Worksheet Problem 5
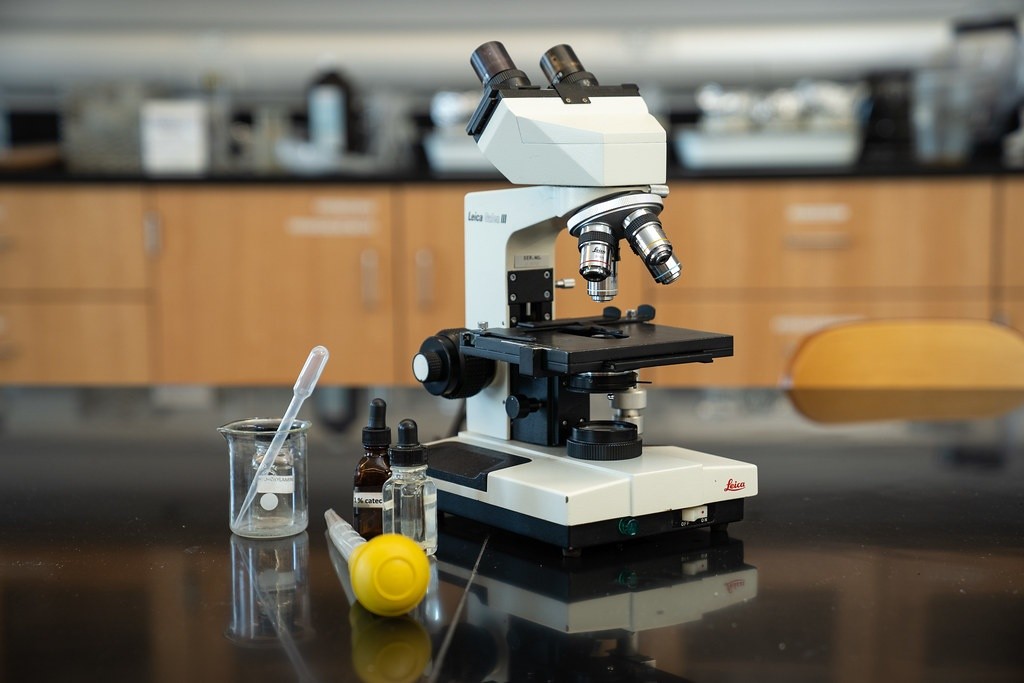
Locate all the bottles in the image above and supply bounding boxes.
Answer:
[353,398,392,539]
[382,419,437,556]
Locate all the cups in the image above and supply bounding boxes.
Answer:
[215,417,312,537]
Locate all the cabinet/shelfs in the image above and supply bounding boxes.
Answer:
[0,175,1024,386]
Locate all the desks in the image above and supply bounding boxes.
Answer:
[0,385,1023,683]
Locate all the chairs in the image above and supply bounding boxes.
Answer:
[786,317,1023,388]
